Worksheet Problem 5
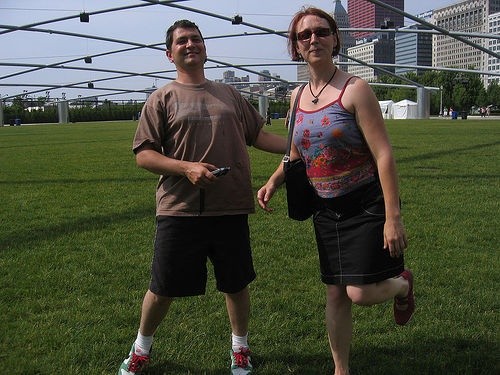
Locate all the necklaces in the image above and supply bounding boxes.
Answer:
[309,66,337,103]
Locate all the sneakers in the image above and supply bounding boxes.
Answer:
[394,271,415,326]
[230,345,253,375]
[118,343,152,375]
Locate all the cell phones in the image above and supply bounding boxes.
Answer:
[211,166,230,176]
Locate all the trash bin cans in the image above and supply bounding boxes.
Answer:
[9,119,14,126]
[72,118,75,123]
[271,113,278,119]
[452,111,457,119]
[461,111,467,119]
[16,119,21,126]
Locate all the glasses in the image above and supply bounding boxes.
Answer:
[297,28,332,40]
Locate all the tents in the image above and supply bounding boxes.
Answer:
[391,99,418,119]
[378,100,393,119]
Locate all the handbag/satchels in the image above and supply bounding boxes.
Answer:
[282,157,317,220]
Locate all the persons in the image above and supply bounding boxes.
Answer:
[449,107,453,117]
[443,106,448,118]
[480,106,490,118]
[116,19,291,375]
[257,7,414,375]
[451,108,457,120]
[137,111,141,120]
[266,108,272,126]
[285,109,290,128]
